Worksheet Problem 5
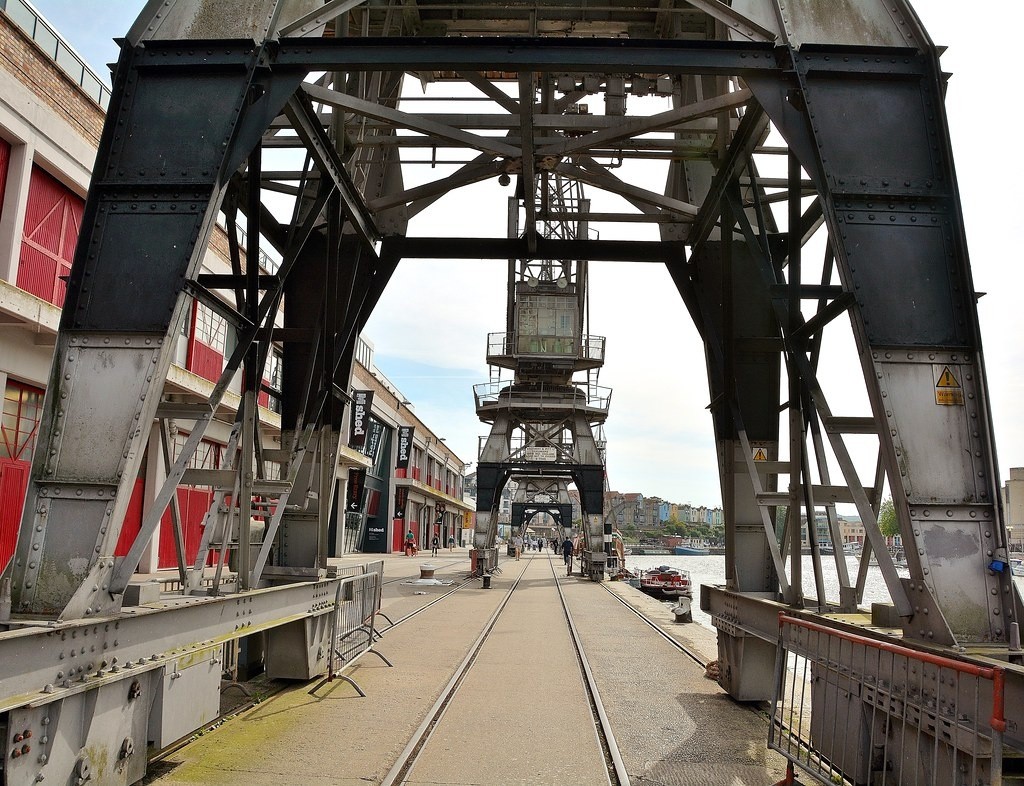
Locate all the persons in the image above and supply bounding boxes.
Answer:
[566,547,573,577]
[524,537,544,552]
[553,537,559,555]
[513,534,523,561]
[497,535,502,550]
[430,532,439,557]
[406,529,414,548]
[562,536,574,566]
[448,535,455,552]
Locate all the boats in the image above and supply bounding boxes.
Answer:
[641,565,692,595]
[673,545,710,556]
